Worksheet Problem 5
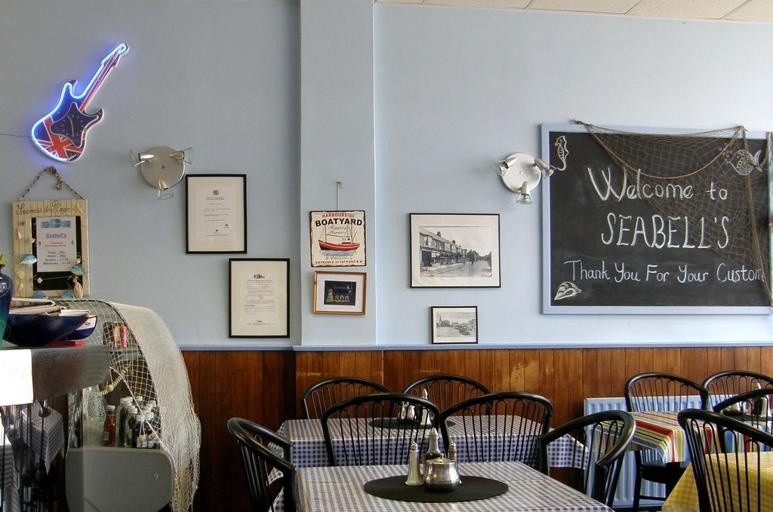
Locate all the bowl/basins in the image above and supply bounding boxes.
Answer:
[2,306,89,349]
[60,315,96,342]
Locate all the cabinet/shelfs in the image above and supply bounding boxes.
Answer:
[53,297,201,510]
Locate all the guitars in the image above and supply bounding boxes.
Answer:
[31,40,128,163]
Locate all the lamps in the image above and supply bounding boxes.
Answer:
[127,145,194,201]
[494,152,554,205]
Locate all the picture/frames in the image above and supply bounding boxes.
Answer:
[428,306,479,344]
[407,210,502,289]
[183,171,248,255]
[227,255,291,338]
[309,208,366,272]
[310,269,366,315]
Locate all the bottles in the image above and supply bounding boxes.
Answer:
[723,382,772,418]
[103,393,162,448]
[0,263,12,350]
[397,387,462,491]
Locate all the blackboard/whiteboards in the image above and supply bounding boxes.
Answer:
[541,122,773,315]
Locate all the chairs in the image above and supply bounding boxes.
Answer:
[401,374,494,415]
[227,417,297,511]
[625,372,709,509]
[426,392,553,469]
[321,392,451,466]
[300,376,402,419]
[0,392,67,512]
[661,370,773,511]
[537,410,636,502]
